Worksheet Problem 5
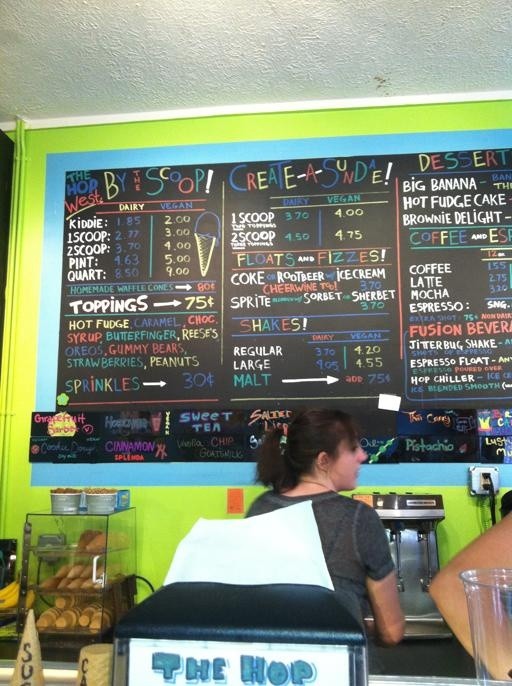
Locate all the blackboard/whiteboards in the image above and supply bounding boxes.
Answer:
[30,149,512,465]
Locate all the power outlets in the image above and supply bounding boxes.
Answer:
[468,465,501,498]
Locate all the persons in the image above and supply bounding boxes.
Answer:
[427,490,512,681]
[245,406,406,647]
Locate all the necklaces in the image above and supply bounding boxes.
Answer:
[300,478,337,492]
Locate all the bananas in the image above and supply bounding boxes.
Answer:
[0,576,36,612]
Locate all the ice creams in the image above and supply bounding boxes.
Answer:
[194,211,221,278]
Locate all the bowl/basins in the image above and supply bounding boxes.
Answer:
[50,491,81,515]
[85,492,118,514]
